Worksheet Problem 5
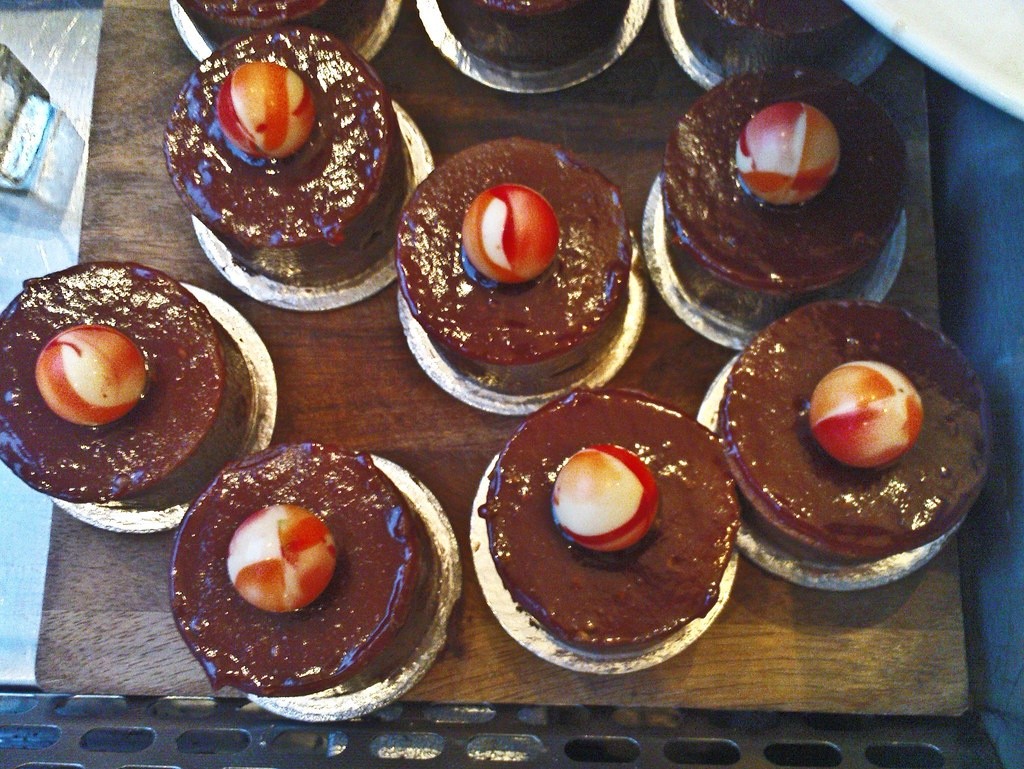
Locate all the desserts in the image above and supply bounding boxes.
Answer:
[0,0,995,723]
